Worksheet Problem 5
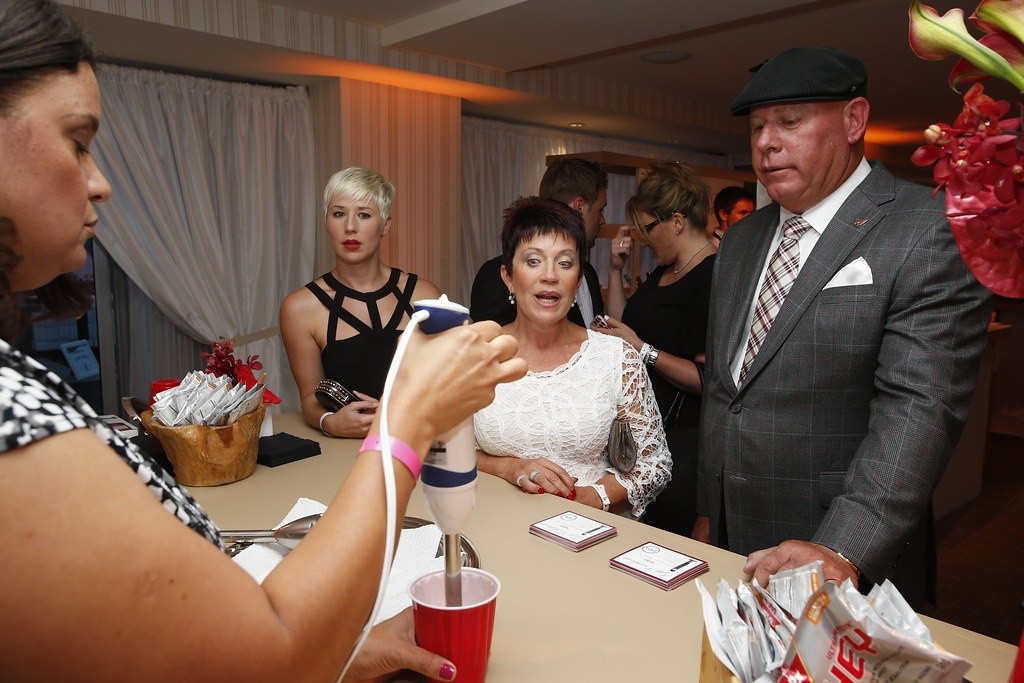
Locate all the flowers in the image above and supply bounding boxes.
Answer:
[908,0,1024,298]
[201,335,262,379]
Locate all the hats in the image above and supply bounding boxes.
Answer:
[730,44,868,117]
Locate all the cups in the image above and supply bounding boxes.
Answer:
[409,566,500,683]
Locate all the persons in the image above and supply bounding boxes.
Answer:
[472,197,673,522]
[691,46,994,613]
[0,0,528,683]
[471,153,609,333]
[609,160,717,524]
[280,166,443,441]
[711,185,759,242]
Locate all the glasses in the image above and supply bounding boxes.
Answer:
[637,210,688,237]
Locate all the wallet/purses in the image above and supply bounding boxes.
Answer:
[315,378,367,415]
[608,419,638,475]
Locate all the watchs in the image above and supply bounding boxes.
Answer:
[646,345,660,368]
[834,549,863,579]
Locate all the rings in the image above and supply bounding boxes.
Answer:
[619,241,623,248]
[516,474,528,486]
[530,470,540,480]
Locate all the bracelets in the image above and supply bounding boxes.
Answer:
[590,482,610,513]
[640,342,651,361]
[358,433,423,488]
[318,412,332,438]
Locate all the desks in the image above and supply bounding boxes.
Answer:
[168,414,1019,683]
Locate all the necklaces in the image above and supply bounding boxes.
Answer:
[673,240,712,274]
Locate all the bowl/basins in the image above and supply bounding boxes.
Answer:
[140,403,266,485]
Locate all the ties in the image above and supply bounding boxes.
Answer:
[736,216,813,394]
